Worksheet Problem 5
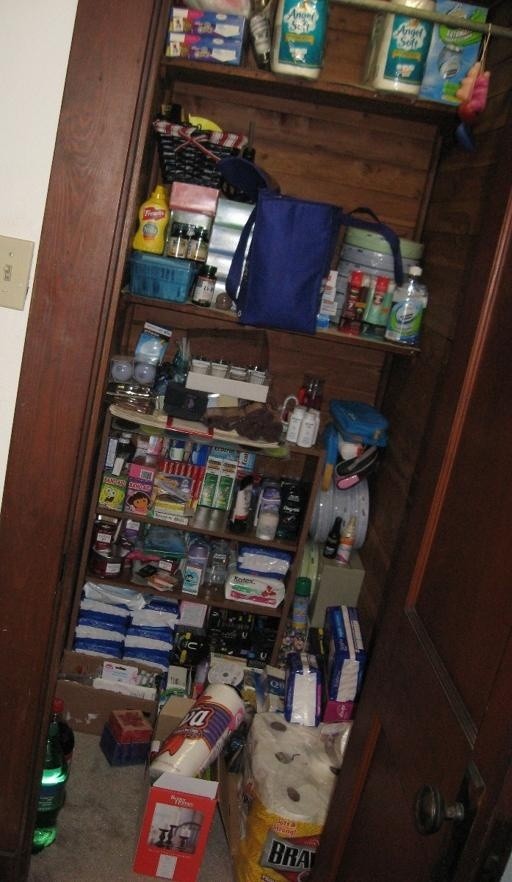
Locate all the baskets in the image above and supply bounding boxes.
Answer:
[153,117,251,191]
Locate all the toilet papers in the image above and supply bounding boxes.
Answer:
[220,713,353,882]
[150,684,246,783]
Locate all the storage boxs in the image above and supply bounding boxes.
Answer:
[133,694,221,882]
[308,550,367,628]
[419,1,489,107]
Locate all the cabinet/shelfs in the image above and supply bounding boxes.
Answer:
[59,405,328,703]
[120,55,476,358]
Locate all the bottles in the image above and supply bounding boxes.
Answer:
[184,225,210,264]
[121,432,135,464]
[285,404,321,449]
[165,221,190,259]
[323,513,358,566]
[255,487,282,541]
[45,697,76,812]
[191,263,217,308]
[185,544,209,585]
[247,1,272,70]
[30,723,69,857]
[115,437,131,464]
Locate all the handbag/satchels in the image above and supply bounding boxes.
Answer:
[225,187,347,336]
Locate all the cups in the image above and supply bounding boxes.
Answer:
[380,263,429,347]
